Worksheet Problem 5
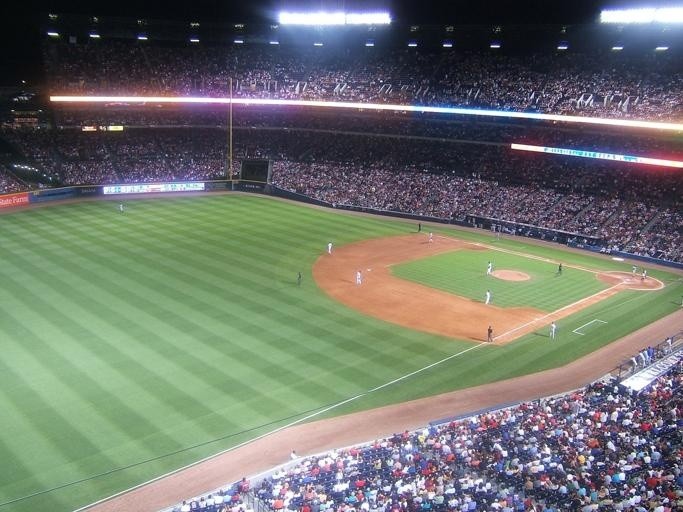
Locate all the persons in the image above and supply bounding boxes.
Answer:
[549,322,556,339]
[487,326,493,342]
[483,290,491,304]
